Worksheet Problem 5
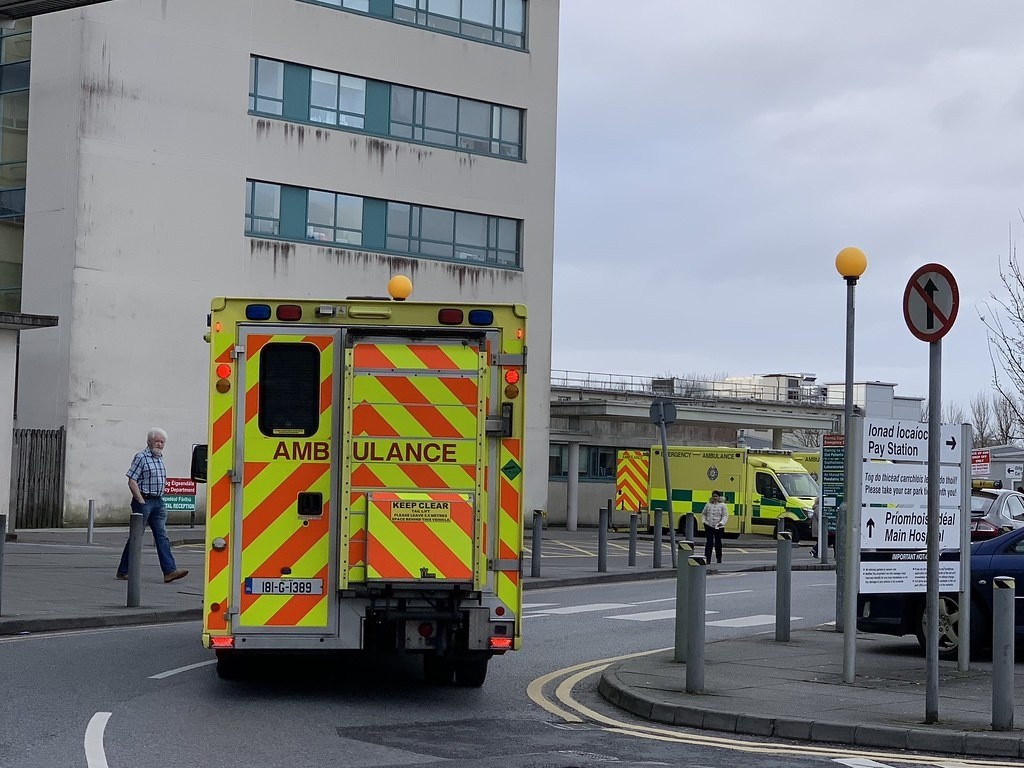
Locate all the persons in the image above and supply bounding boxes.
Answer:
[114,427,189,584]
[701,490,728,565]
[808,496,819,558]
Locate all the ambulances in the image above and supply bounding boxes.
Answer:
[616,446,819,543]
[202,275,530,691]
[791,453,820,487]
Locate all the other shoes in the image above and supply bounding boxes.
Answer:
[707,562,710,564]
[116,573,128,580]
[809,550,818,558]
[164,570,189,583]
[717,559,721,563]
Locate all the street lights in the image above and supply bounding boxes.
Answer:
[835,245,868,683]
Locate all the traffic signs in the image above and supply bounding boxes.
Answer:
[860,507,960,549]
[1006,464,1023,478]
[863,417,962,463]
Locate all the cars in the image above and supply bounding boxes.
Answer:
[857,526,1024,661]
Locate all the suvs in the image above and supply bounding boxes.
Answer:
[971,479,1024,542]
